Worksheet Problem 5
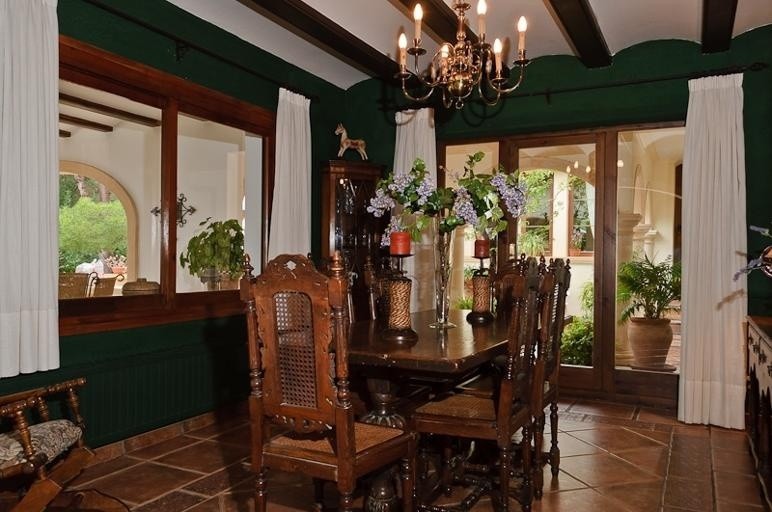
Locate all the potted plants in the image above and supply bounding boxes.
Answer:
[616,255,682,370]
[180,215,243,291]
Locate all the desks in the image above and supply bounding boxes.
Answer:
[320,308,571,509]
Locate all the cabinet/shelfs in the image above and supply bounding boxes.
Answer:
[322,160,388,269]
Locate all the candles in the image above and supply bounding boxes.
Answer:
[389,232,411,255]
[475,239,490,256]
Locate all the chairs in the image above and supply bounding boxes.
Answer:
[59,269,125,299]
[408,253,555,510]
[1,378,97,511]
[242,252,417,510]
[454,257,571,500]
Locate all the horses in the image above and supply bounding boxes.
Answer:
[334,122,368,160]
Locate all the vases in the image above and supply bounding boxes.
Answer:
[427,231,460,328]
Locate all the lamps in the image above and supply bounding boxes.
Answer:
[396,0,531,111]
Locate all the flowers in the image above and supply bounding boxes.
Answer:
[364,150,531,315]
